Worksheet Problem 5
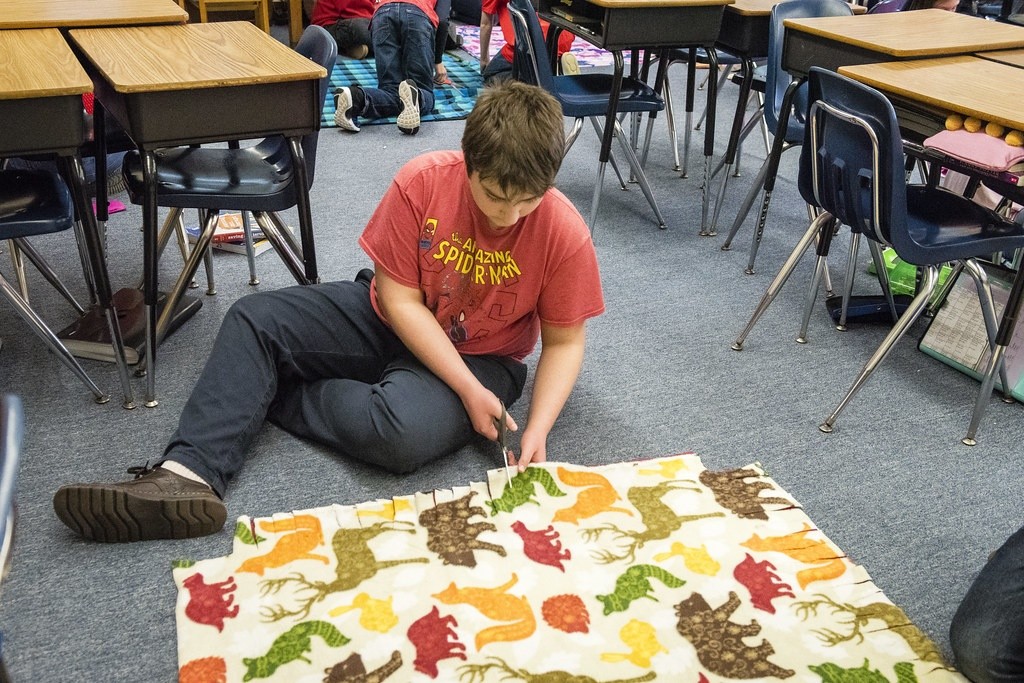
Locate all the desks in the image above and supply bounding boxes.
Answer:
[534,2,736,236]
[746,7,1024,273]
[0,29,133,410]
[191,0,271,34]
[710,0,867,234]
[838,54,1024,329]
[976,48,1024,69]
[69,21,327,408]
[0,2,189,27]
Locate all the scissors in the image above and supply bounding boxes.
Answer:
[433,75,466,96]
[491,401,514,489]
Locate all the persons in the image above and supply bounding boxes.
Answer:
[311,1,377,59]
[331,0,451,137]
[901,0,963,13]
[480,0,583,84]
[5,146,135,199]
[53,76,605,542]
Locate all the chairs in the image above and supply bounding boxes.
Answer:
[123,26,337,297]
[867,0,906,13]
[508,0,668,239]
[635,42,771,178]
[0,166,108,404]
[734,68,1024,433]
[723,1,854,253]
[23,102,133,156]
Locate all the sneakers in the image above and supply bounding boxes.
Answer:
[347,44,369,59]
[397,79,420,136]
[334,87,360,131]
[558,52,579,75]
[53,467,226,543]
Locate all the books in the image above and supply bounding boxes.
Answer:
[185,211,294,258]
[53,287,204,364]
